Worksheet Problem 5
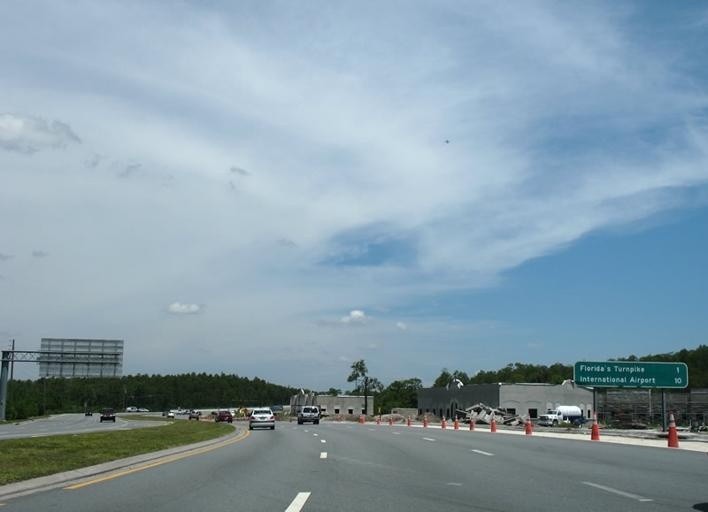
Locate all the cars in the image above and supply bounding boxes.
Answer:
[248,408,275,430]
[100,407,115,423]
[168,411,174,418]
[215,411,233,423]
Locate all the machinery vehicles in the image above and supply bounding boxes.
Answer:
[537,405,583,428]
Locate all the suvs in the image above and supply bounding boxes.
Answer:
[297,405,320,424]
[189,410,199,421]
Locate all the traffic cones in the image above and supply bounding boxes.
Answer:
[667,411,681,448]
[470,416,475,430]
[525,414,534,435]
[454,414,459,431]
[590,411,600,441]
[441,414,447,430]
[423,414,428,428]
[490,413,497,432]
[358,412,412,427]
